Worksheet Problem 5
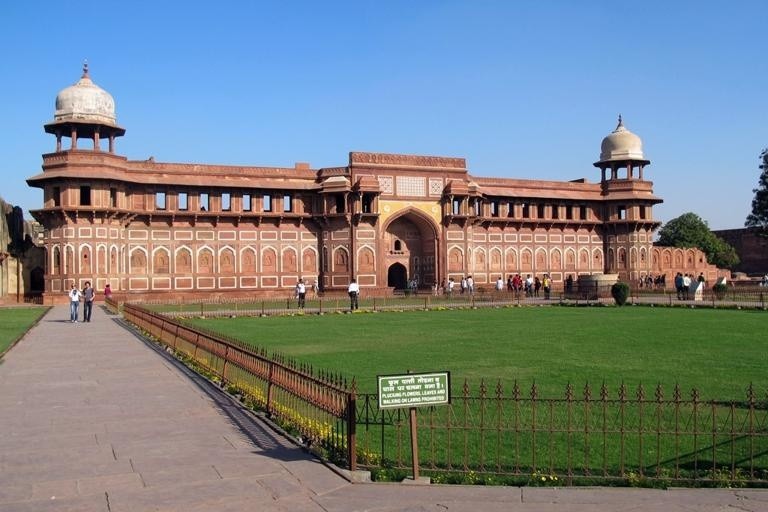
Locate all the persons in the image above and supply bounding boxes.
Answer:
[296,279,306,309]
[103,283,111,307]
[759,274,768,288]
[82,282,96,323]
[68,283,83,323]
[431,275,473,297]
[638,271,707,300]
[494,273,552,300]
[312,280,319,299]
[566,275,574,300]
[348,278,360,310]
[403,278,419,297]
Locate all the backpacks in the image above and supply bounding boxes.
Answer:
[537,282,541,288]
[350,291,356,296]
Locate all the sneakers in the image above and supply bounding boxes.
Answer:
[84,319,91,322]
[71,320,78,323]
